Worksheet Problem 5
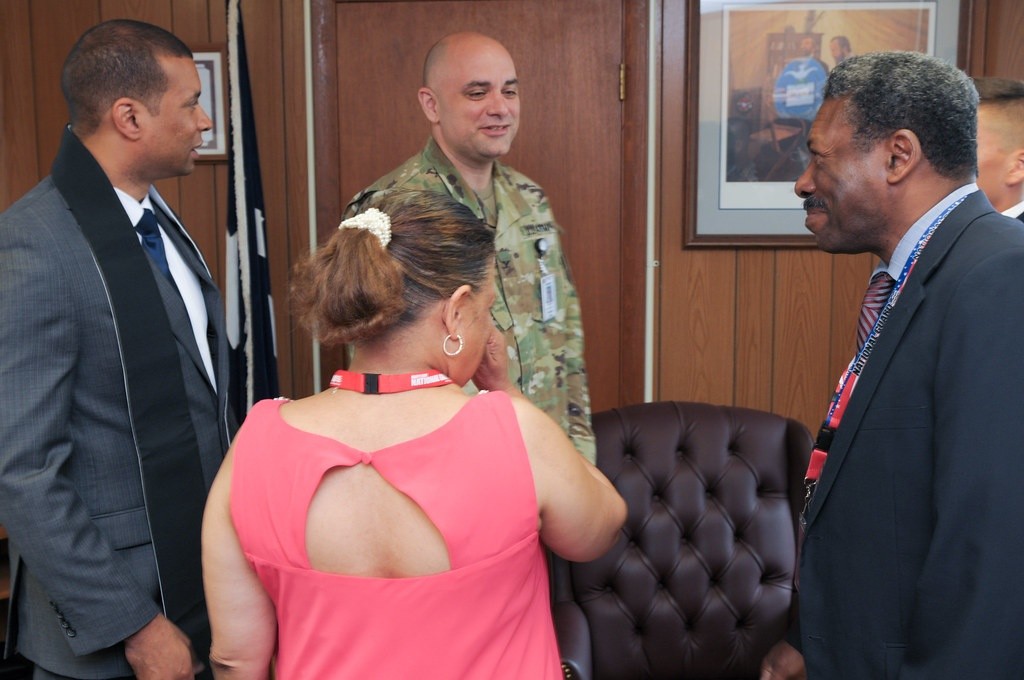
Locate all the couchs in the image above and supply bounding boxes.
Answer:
[543,400,815,680]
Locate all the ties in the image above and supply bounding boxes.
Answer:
[136,207,180,295]
[856,271,897,358]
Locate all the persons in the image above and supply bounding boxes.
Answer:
[0,17,240,680]
[965,76,1024,224]
[758,50,1024,680]
[200,186,628,680]
[341,29,597,474]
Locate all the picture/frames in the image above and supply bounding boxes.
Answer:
[190,42,229,164]
[684,0,975,251]
